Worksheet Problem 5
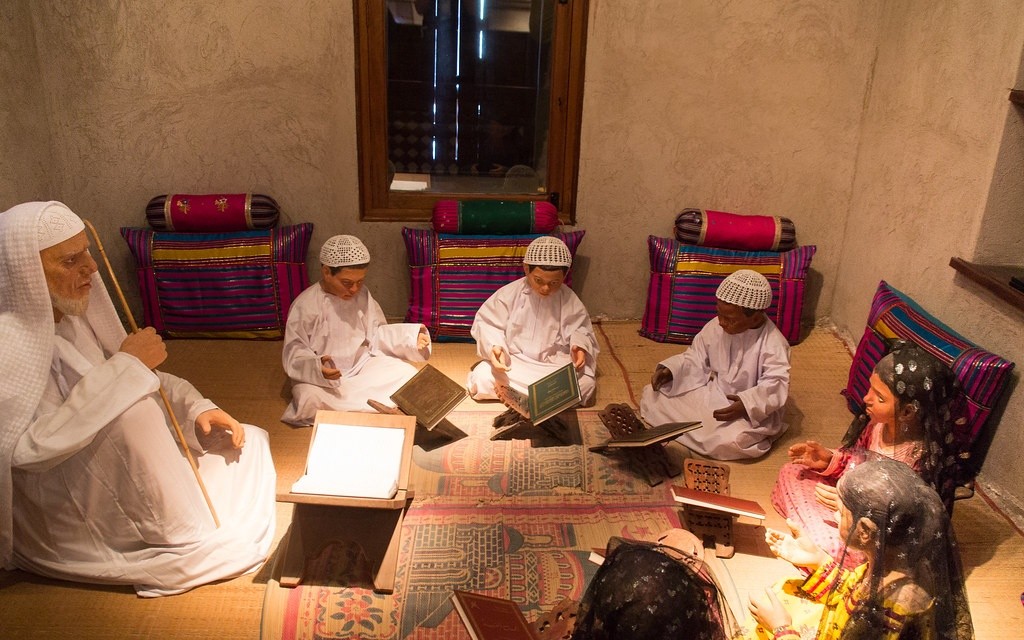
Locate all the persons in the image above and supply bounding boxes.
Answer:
[0,200,277,599]
[639,268,792,461]
[466,235,601,409]
[280,233,432,428]
[571,535,743,640]
[746,338,977,640]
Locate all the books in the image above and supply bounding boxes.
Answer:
[289,423,406,500]
[528,363,583,427]
[389,363,469,432]
[607,421,703,447]
[670,484,767,520]
